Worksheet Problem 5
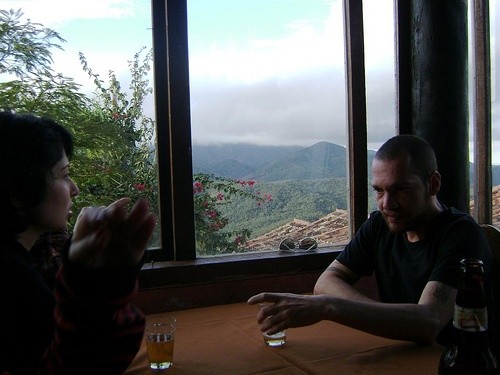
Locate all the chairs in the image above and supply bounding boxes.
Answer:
[480,224,500,371]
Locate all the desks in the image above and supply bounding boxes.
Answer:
[124,292,447,375]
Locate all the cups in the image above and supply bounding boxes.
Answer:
[145,314,177,369]
[258,302,286,346]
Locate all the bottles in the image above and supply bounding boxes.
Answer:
[438,258,497,375]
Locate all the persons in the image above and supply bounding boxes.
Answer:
[247,134,500,375]
[0,110,158,375]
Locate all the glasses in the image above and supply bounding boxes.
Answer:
[279,237,318,253]
